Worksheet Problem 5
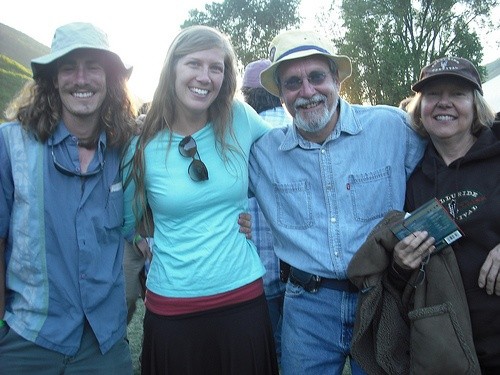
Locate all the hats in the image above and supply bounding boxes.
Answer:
[242,59,272,87]
[412,58,483,97]
[31,22,133,81]
[260,31,353,98]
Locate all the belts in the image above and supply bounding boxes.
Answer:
[288,266,359,294]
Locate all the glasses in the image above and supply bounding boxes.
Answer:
[393,221,431,291]
[178,135,210,183]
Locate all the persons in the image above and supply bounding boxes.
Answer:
[118,114,167,327]
[115,26,280,375]
[246,28,432,375]
[240,58,294,373]
[385,56,500,375]
[1,21,142,375]
